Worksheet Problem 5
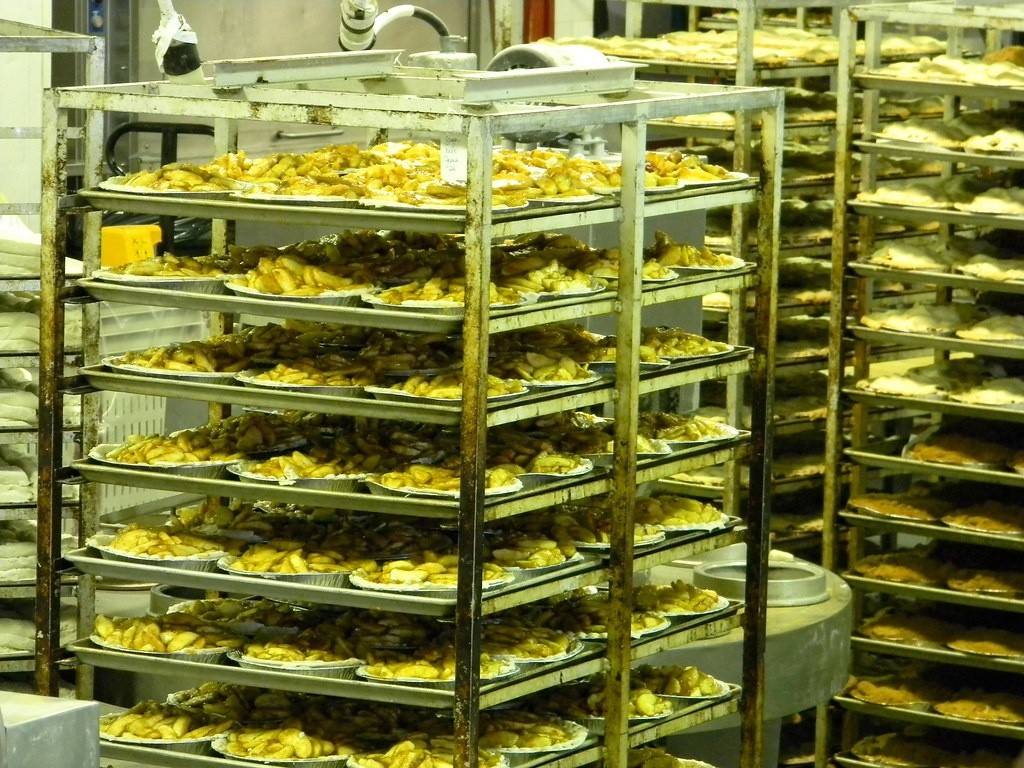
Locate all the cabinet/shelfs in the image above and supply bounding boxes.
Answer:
[492,0,1024,767]
[0,16,108,700]
[31,46,788,768]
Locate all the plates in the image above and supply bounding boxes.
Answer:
[87,0,1024,768]
